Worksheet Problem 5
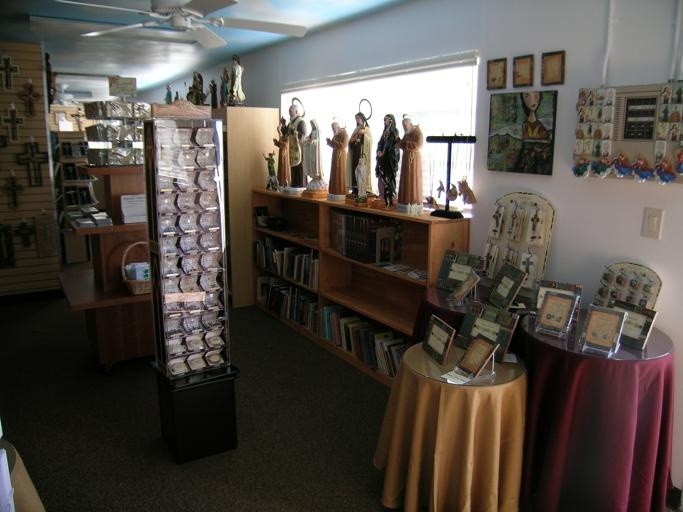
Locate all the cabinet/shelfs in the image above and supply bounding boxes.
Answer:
[250,187,470,389]
[57,107,279,367]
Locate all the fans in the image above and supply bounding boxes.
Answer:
[52,0,307,49]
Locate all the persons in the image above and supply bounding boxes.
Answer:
[519,92,551,140]
[163,55,245,107]
[263,105,423,214]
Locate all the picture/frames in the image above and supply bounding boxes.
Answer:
[513,54,533,87]
[487,57,506,89]
[425,250,657,378]
[542,50,565,84]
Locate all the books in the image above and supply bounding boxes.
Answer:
[255,210,408,379]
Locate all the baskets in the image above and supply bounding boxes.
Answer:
[121,240,153,296]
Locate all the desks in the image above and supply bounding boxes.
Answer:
[372,341,528,512]
[520,302,675,512]
